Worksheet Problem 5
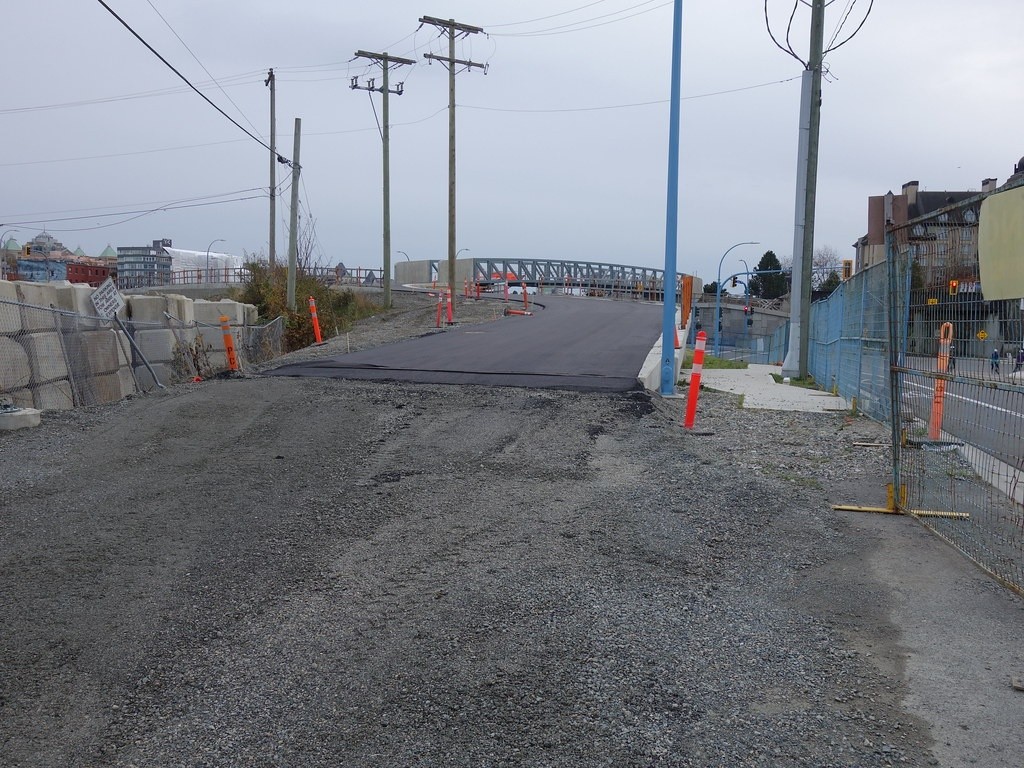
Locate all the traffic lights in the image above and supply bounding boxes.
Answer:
[744,306,747,313]
[732,276,737,287]
[750,307,754,315]
[950,280,959,295]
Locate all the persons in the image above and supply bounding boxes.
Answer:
[1013,350,1024,373]
[989,348,1000,375]
[947,346,956,372]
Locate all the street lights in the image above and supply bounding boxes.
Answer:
[207,238,226,283]
[0,229,23,257]
[4,239,18,280]
[714,241,761,358]
